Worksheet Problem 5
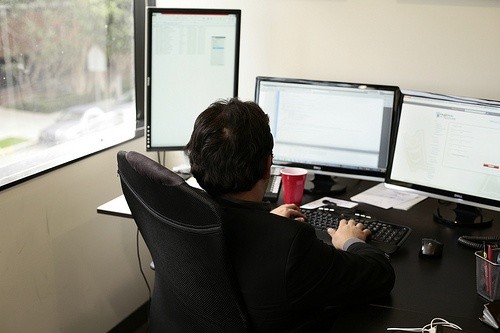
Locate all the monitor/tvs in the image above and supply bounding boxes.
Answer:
[144,8,241,152]
[384,90,500,229]
[254,77,400,195]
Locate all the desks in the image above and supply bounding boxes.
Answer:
[97,176,500,333]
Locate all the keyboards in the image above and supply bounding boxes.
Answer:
[291,208,411,255]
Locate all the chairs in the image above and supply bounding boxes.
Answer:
[117,149,253,333]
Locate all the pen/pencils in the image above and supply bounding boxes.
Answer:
[487,244,493,299]
[482,240,489,295]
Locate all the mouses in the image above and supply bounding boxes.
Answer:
[420,239,444,260]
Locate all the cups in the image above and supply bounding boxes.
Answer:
[280,167,308,206]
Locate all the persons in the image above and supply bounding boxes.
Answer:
[182,97,395,333]
